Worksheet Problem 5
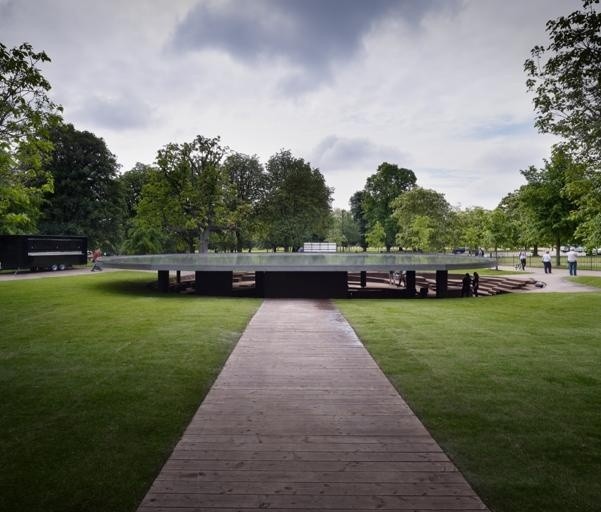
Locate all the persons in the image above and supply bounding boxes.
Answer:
[519,248,527,271]
[461,273,472,297]
[472,272,480,297]
[389,270,407,289]
[90,246,103,272]
[475,247,484,256]
[566,246,580,277]
[542,250,552,274]
